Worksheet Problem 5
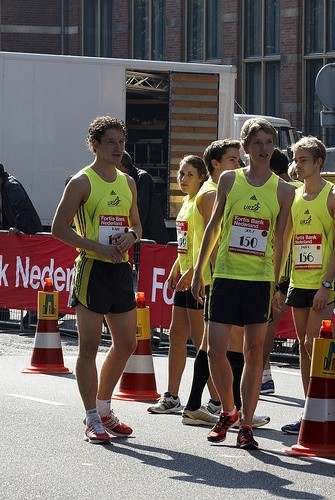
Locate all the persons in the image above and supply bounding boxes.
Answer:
[0,163,42,236]
[259,150,304,393]
[147,156,221,414]
[117,150,170,244]
[190,117,295,447]
[181,138,270,428]
[51,117,142,443]
[279,136,335,434]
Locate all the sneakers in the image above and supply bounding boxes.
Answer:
[237,427,259,449]
[281,418,301,434]
[202,403,223,417]
[182,407,219,425]
[101,413,132,437]
[260,380,275,393]
[207,407,239,442]
[232,411,269,427]
[84,419,110,443]
[148,397,182,412]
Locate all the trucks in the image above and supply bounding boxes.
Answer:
[0,51,301,247]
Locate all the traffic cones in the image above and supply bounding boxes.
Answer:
[19,278,74,375]
[283,320,335,460]
[112,293,162,402]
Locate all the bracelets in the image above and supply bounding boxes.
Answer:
[129,231,138,243]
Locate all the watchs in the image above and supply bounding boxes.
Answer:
[322,281,332,288]
